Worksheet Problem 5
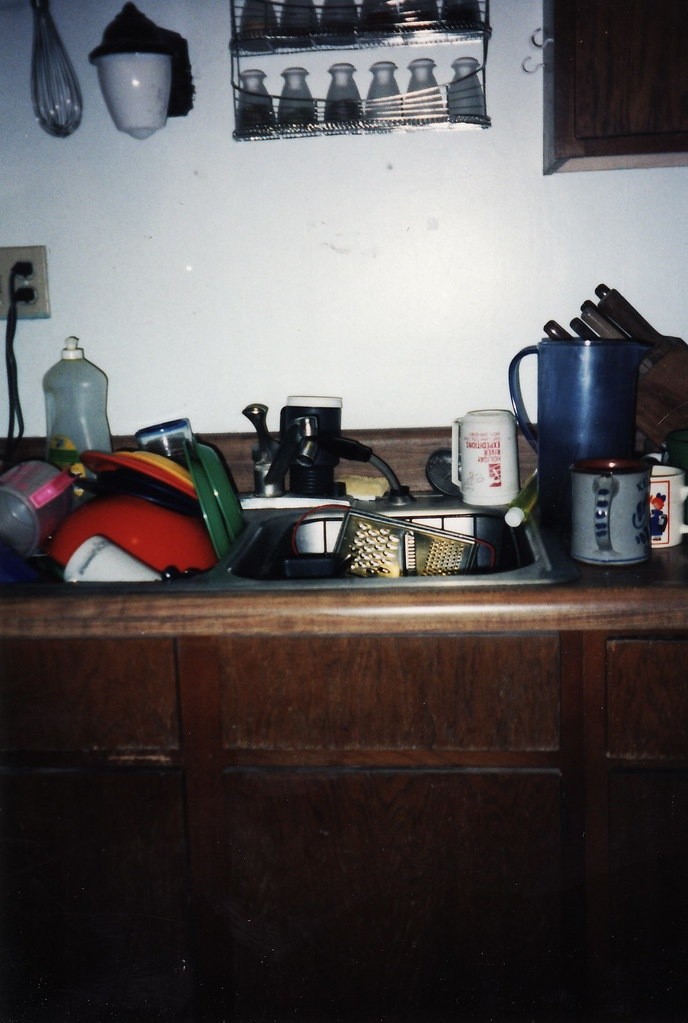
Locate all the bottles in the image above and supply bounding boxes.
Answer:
[43,337,112,471]
[237,2,487,129]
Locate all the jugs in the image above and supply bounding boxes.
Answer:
[508,339,655,568]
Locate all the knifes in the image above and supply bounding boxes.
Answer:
[543,283,671,375]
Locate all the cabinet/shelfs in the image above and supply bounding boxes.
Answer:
[542,0,688,175]
[228,0,492,140]
[0,630,688,1023]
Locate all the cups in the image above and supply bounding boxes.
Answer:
[62,535,162,581]
[0,461,75,559]
[567,456,654,566]
[136,417,196,472]
[279,396,343,500]
[92,51,172,140]
[451,408,519,506]
[647,464,688,548]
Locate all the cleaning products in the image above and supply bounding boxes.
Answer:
[41,335,114,472]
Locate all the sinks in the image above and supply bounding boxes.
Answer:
[0,520,248,582]
[227,510,534,579]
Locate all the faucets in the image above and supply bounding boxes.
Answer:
[242,403,321,498]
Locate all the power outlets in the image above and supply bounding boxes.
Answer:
[0,244,54,320]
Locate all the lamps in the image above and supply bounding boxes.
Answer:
[88,2,196,140]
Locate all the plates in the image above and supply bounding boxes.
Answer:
[79,448,198,502]
[182,438,244,560]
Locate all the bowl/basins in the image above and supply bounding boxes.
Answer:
[46,492,217,574]
